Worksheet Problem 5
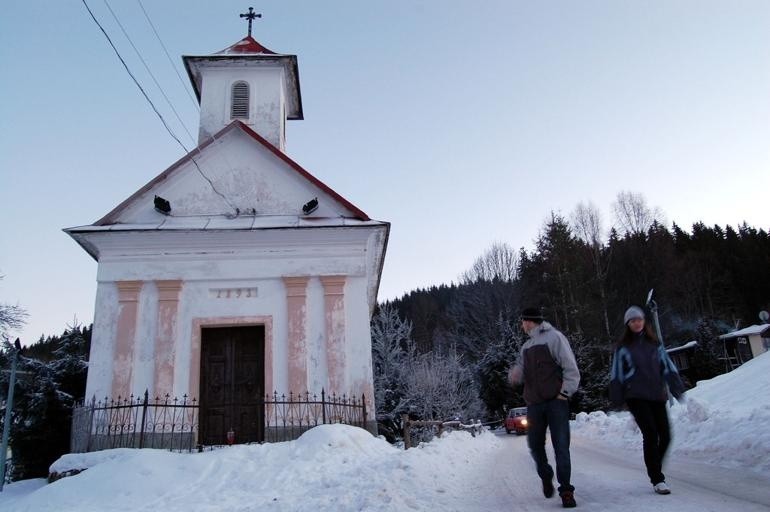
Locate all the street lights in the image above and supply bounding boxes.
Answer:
[646,288,675,407]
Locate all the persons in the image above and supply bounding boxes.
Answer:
[510,306,582,508]
[609,306,687,495]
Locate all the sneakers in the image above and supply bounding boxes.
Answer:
[559,491,577,508]
[651,481,671,494]
[541,470,555,499]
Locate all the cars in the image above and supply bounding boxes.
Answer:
[505,406,528,435]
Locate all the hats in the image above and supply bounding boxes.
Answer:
[622,304,648,327]
[519,307,543,322]
